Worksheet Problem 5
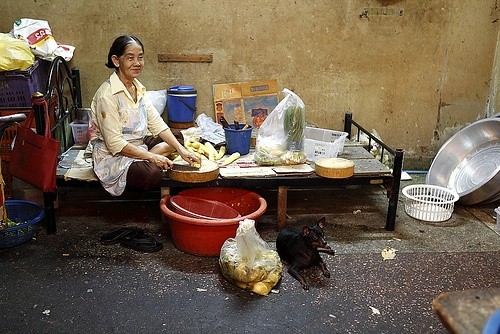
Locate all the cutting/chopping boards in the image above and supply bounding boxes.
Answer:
[315,157,355,179]
[167,160,220,183]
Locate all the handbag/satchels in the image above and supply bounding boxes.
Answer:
[255,88,308,166]
[219,219,284,295]
[7,101,60,193]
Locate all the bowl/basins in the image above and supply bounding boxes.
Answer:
[160,186,268,256]
[425,118,500,207]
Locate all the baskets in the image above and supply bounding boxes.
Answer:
[1,110,38,200]
[401,184,459,223]
[69,107,91,143]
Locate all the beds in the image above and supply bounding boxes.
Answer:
[31,67,405,234]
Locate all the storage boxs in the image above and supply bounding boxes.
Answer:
[0,107,33,161]
[70,117,92,144]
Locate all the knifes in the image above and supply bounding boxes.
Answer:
[167,164,199,171]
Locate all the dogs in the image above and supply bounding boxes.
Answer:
[276,216,335,291]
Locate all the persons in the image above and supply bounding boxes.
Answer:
[90,35,201,196]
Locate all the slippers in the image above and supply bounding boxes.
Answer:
[121,233,163,253]
[101,226,144,246]
